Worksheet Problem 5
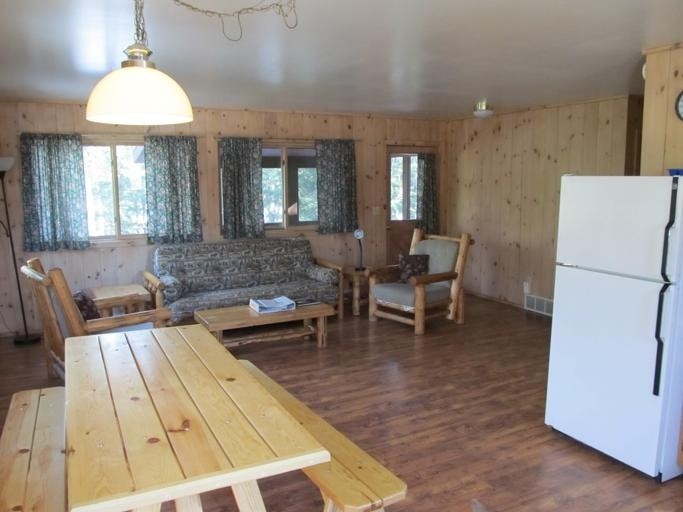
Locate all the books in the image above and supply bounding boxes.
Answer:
[293,297,322,307]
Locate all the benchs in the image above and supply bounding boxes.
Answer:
[0,387,66,512]
[238,357,408,512]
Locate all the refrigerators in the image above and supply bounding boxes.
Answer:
[545,176,683,481]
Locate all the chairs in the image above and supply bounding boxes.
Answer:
[20,257,172,385]
[364,228,470,335]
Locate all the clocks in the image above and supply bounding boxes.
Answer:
[674,88,682,121]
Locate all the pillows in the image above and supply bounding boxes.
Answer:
[397,253,429,283]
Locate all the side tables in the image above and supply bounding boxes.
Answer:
[342,265,369,316]
[91,283,151,317]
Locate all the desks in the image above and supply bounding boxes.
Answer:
[63,322,333,512]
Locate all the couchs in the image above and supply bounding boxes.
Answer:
[142,237,343,328]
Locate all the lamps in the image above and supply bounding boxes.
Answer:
[473,102,494,119]
[353,228,366,272]
[82,1,195,127]
[0,154,42,348]
